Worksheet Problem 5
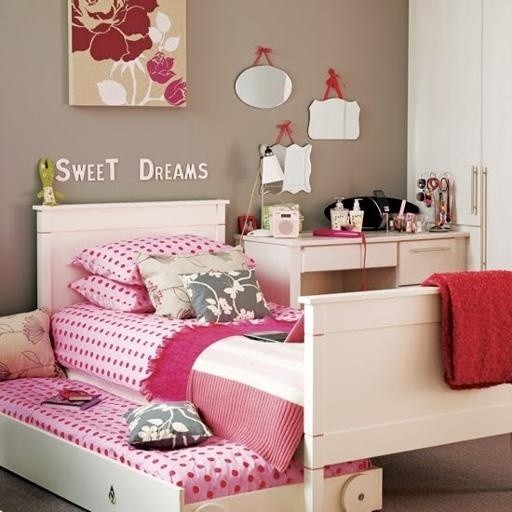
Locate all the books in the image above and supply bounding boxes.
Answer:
[39,394,104,412]
[59,388,93,402]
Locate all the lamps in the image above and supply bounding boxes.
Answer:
[249,146,285,236]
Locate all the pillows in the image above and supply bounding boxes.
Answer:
[66,235,272,321]
[0,307,66,378]
[122,400,207,448]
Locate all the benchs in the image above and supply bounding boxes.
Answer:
[0,379,383,512]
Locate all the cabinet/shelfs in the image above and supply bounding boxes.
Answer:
[235,231,468,308]
[407,0,511,271]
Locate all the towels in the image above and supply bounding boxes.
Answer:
[422,270,512,389]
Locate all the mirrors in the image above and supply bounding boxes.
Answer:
[260,145,312,193]
[234,65,292,108]
[307,99,360,141]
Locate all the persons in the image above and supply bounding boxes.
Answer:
[247,220,254,232]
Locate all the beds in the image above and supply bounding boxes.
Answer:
[33,200,511,512]
